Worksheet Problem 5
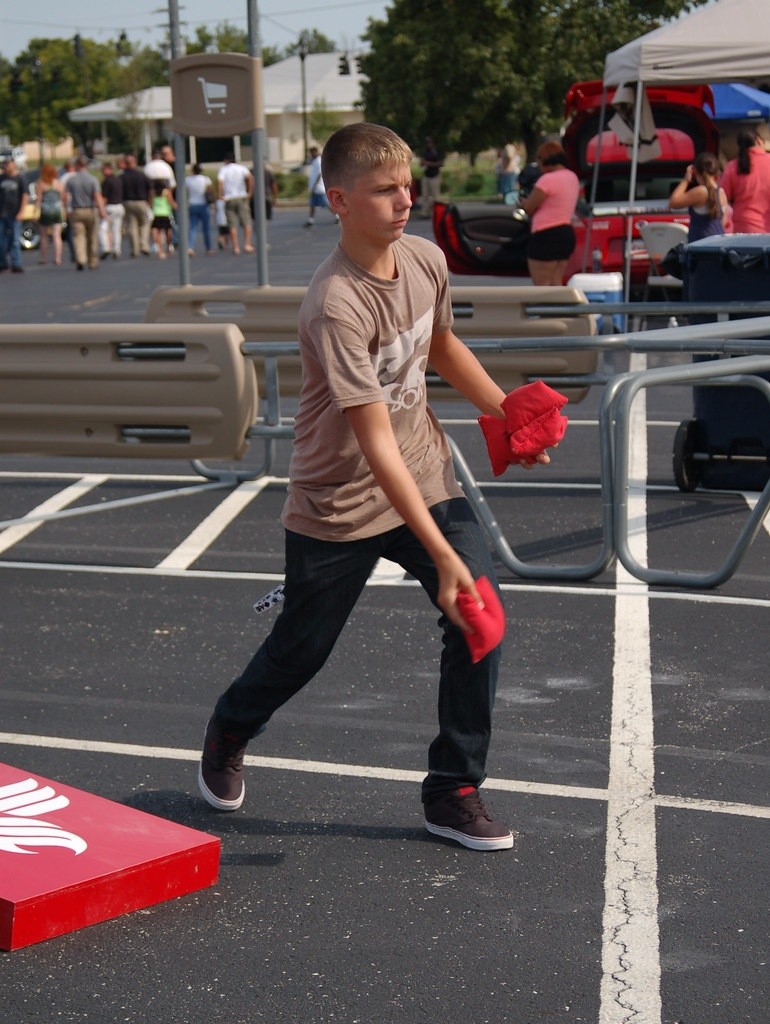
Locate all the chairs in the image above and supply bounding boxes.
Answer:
[638,222,699,331]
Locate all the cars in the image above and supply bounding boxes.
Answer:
[432,80,722,298]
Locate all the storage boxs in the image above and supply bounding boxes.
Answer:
[569,272,625,335]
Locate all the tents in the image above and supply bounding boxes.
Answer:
[584,0,770,332]
[703,83,770,121]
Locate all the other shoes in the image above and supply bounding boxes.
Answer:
[302,220,313,227]
[11,265,24,274]
[334,218,341,224]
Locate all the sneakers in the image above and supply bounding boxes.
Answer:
[197,710,248,810]
[424,772,514,850]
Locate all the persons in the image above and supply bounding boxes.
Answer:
[197,123,559,848]
[409,134,442,219]
[302,147,341,228]
[668,153,727,327]
[520,143,581,287]
[0,146,277,274]
[494,135,520,199]
[720,128,770,233]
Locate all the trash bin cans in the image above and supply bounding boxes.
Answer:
[660,233,770,493]
